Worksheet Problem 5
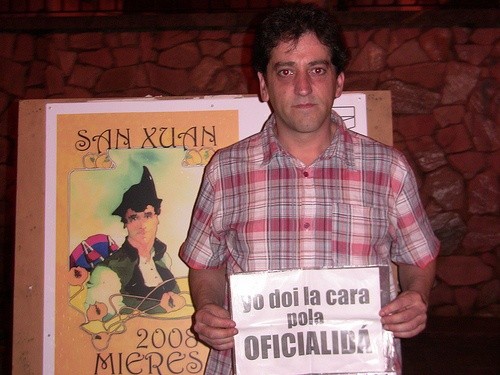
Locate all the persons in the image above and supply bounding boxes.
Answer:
[179,7,441,375]
